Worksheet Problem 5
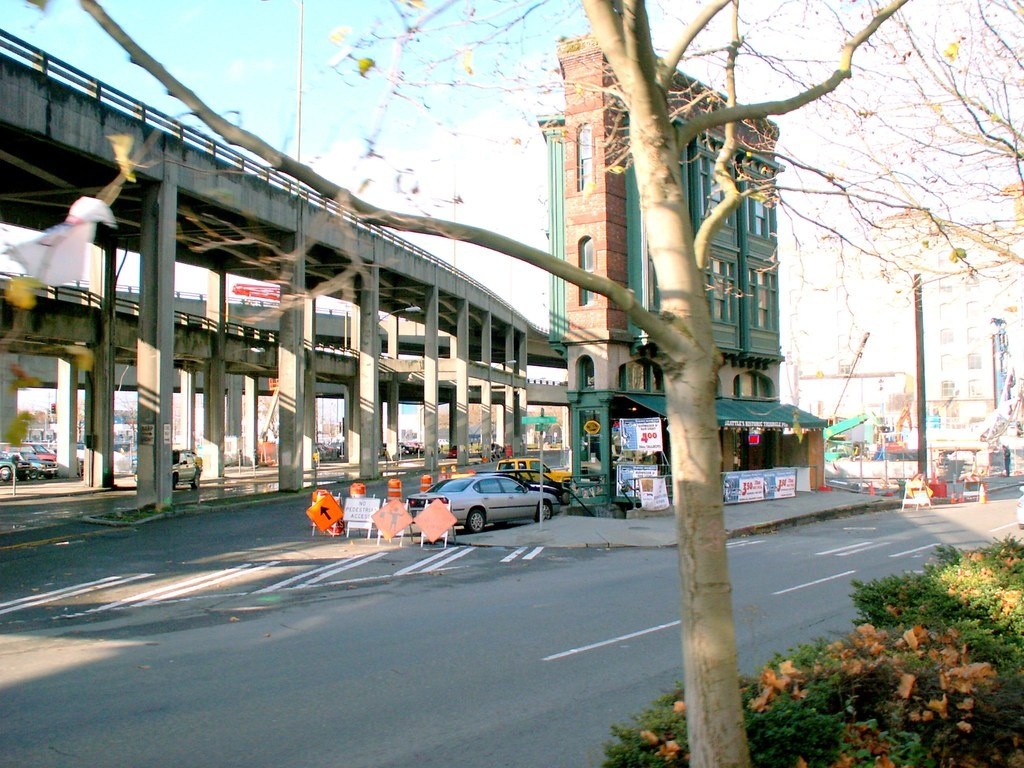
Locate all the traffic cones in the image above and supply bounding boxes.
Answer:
[957,490,964,503]
[978,483,987,505]
[950,490,956,504]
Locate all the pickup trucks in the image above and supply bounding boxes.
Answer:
[450,458,572,483]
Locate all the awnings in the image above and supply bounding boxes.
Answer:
[626,395,829,431]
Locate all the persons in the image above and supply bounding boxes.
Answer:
[1002,446,1011,477]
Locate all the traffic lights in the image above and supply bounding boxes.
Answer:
[51,404,56,413]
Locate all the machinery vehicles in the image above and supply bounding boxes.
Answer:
[821,411,890,462]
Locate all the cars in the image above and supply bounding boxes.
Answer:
[480,443,501,458]
[398,442,441,455]
[0,443,85,482]
[1016,495,1024,530]
[406,476,560,534]
[170,449,203,490]
[448,445,457,458]
[316,442,341,461]
[475,468,571,506]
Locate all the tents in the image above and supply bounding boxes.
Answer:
[930,441,984,478]
